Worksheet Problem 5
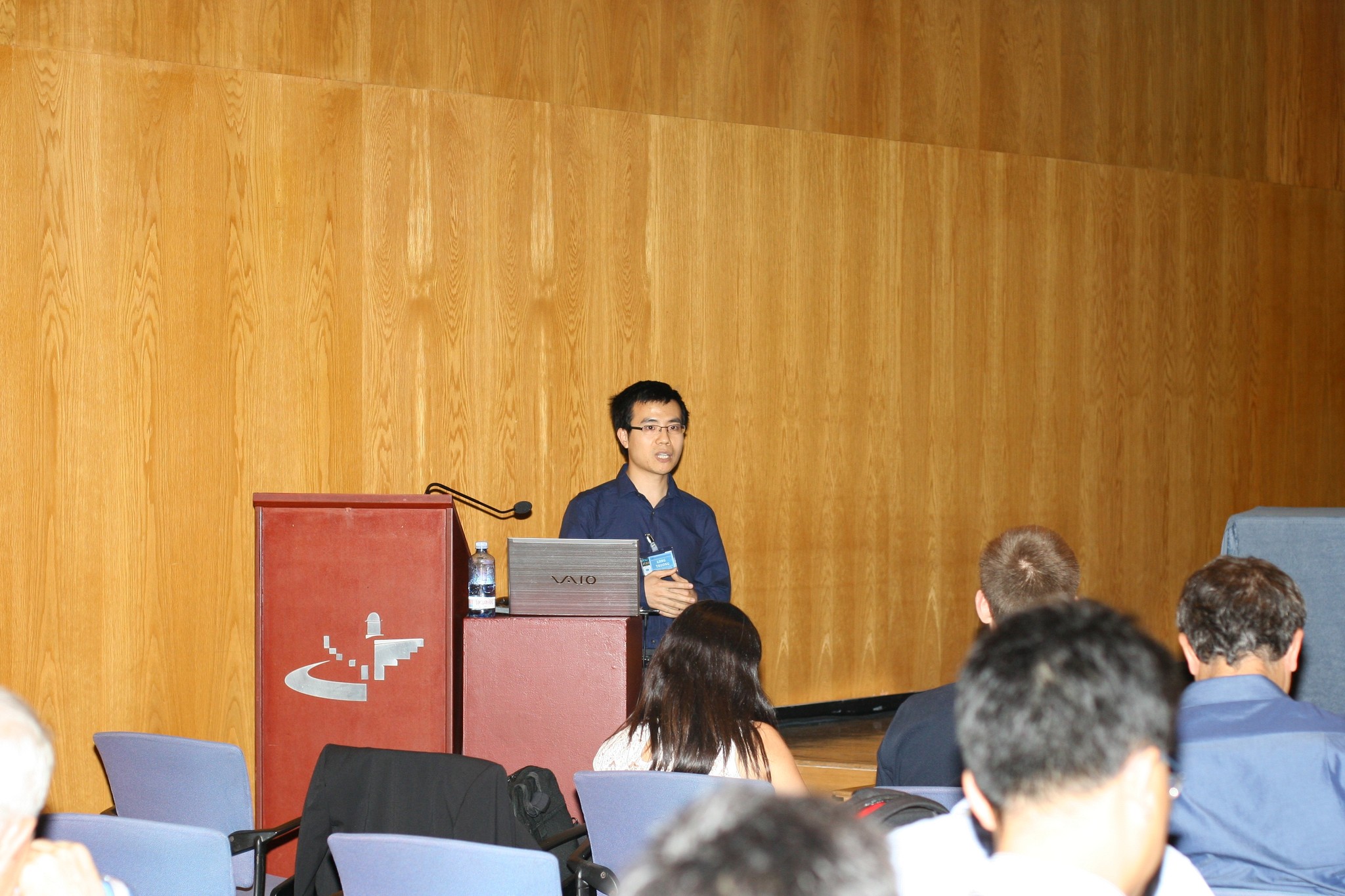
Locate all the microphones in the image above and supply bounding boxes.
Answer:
[425,482,532,515]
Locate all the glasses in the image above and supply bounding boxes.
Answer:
[626,424,686,435]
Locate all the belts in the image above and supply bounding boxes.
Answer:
[642,656,653,669]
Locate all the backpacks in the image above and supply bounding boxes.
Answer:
[509,765,595,887]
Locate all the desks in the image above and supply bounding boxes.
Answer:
[1222,506,1344,723]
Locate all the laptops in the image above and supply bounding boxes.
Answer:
[506,537,641,617]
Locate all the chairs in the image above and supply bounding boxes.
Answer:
[92,731,300,896]
[323,743,590,896]
[35,812,236,896]
[566,767,775,895]
[326,831,565,896]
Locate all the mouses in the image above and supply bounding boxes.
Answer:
[496,596,508,607]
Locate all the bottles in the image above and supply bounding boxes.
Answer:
[467,541,497,618]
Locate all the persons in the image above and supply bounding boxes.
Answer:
[1144,553,1345,896]
[884,796,1218,896]
[0,686,131,896]
[559,379,732,679]
[874,525,1089,789]
[910,591,1193,896]
[597,786,900,896]
[592,598,813,798]
